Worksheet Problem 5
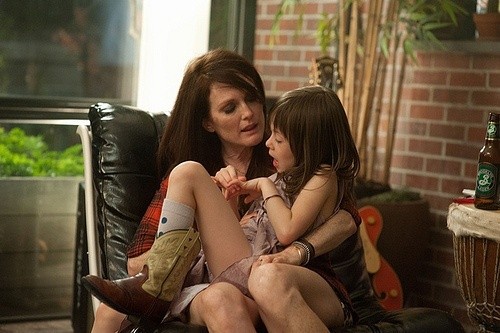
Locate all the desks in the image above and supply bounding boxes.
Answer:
[447,196,500,333]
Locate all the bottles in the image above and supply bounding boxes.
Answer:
[474,112,500,210]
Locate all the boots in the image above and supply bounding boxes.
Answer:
[82,226,201,328]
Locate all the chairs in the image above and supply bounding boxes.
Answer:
[72,95,466,333]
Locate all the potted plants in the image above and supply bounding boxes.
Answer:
[0,127,84,315]
[356,190,431,308]
[377,0,500,68]
[268,0,471,301]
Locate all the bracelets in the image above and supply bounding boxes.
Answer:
[261,194,284,209]
[292,239,315,266]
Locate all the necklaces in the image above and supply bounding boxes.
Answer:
[224,160,246,174]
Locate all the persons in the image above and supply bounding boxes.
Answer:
[125,49,362,333]
[81,86,359,333]
